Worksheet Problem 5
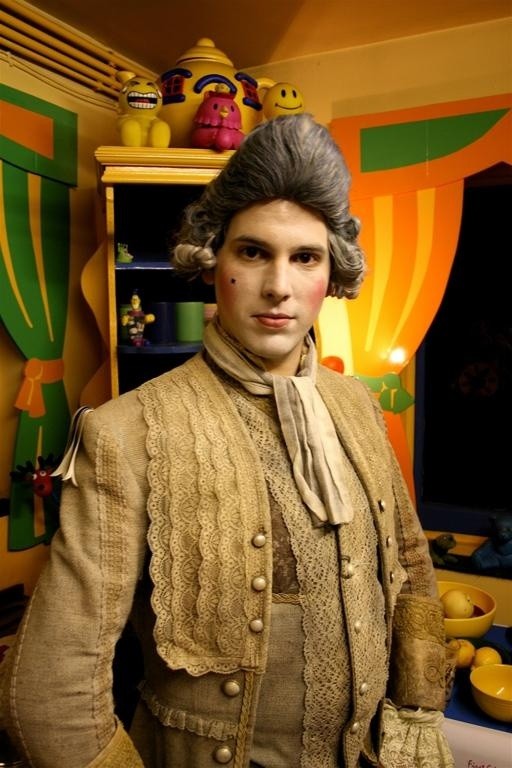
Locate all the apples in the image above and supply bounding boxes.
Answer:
[471,647,502,670]
[439,588,474,619]
[447,639,475,668]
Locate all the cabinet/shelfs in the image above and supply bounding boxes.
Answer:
[93,143,323,398]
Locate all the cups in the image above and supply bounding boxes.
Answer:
[176,302,207,342]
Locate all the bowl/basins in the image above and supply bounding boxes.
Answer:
[470,664,512,721]
[436,581,497,640]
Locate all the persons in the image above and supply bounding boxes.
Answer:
[121,293,155,348]
[0,113,455,768]
